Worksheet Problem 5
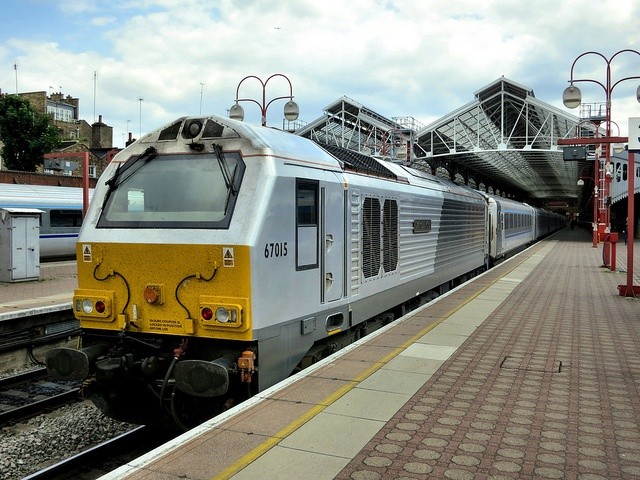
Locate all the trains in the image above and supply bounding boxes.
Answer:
[0,182,95,257]
[45,113,568,432]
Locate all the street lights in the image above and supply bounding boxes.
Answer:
[576,165,614,243]
[361,128,407,162]
[229,73,299,127]
[562,49,640,267]
[575,120,625,248]
